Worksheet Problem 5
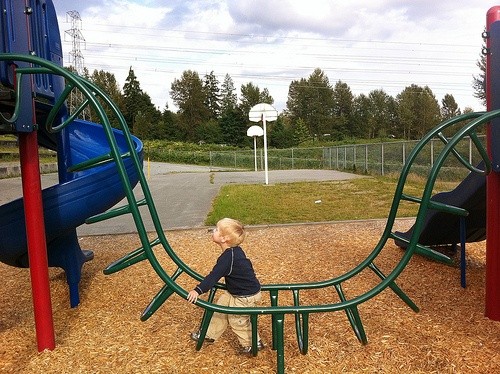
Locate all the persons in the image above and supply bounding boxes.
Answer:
[186,218,265,354]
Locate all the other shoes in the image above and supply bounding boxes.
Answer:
[190,332,214,342]
[246,340,264,353]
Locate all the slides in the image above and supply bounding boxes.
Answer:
[0,108,150,268]
[394,159,487,249]
